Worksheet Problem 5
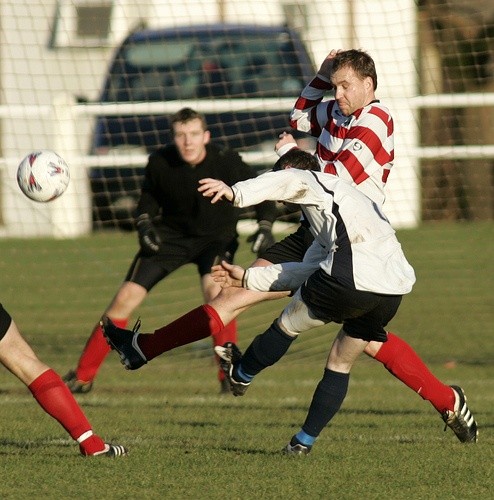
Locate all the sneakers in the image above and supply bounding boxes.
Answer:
[94,442,128,458]
[443,384,478,446]
[100,313,149,371]
[63,370,92,394]
[213,339,251,397]
[282,443,310,457]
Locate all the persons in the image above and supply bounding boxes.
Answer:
[1,303,127,457]
[99,47,480,445]
[63,107,275,394]
[197,151,416,456]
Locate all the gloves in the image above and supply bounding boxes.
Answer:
[247,220,274,256]
[135,213,162,253]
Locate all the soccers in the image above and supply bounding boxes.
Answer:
[17,149,70,202]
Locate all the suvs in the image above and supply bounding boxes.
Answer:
[77,19,328,234]
